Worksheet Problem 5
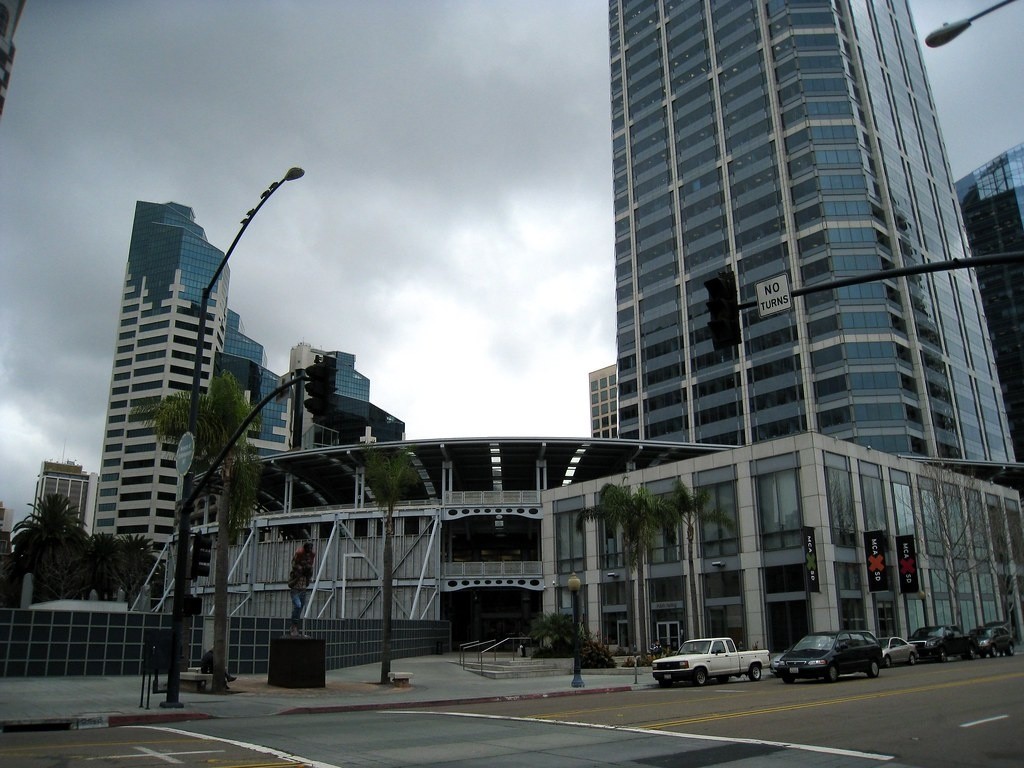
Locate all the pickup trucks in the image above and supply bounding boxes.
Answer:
[652,637,770,688]
[907,625,978,663]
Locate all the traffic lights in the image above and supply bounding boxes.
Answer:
[704,271,742,351]
[186,597,201,615]
[304,363,339,416]
[191,531,212,578]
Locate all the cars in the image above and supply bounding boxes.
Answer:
[770,641,832,678]
[876,637,919,668]
[968,626,1015,658]
[778,630,882,684]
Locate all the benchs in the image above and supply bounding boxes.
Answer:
[180,672,213,691]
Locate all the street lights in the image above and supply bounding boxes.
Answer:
[568,572,585,688]
[159,167,304,710]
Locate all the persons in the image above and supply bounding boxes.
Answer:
[651,640,662,655]
[201,645,237,690]
[289,543,315,636]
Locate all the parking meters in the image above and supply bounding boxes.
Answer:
[633,647,638,684]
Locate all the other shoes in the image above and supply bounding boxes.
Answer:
[225,685,230,689]
[227,675,237,682]
